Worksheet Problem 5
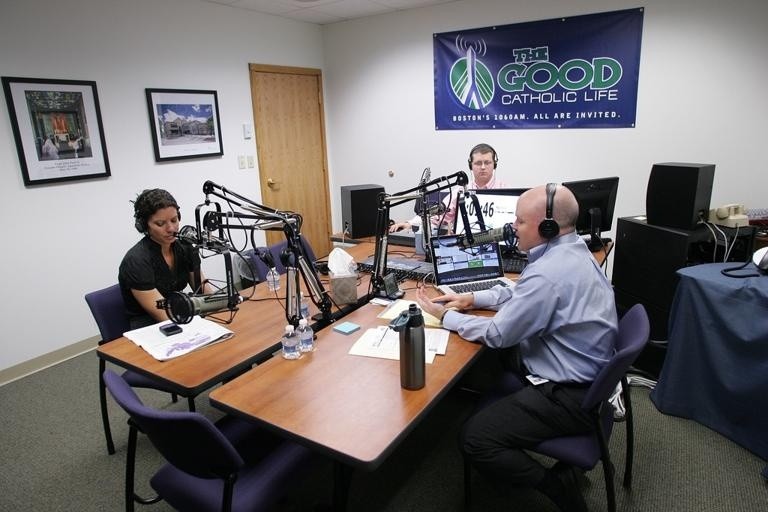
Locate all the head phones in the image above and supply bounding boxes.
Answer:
[538,183,559,240]
[312,261,329,275]
[135,188,181,233]
[251,215,298,269]
[468,144,498,170]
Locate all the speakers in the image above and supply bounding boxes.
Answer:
[646,162,716,233]
[612,215,756,310]
[612,288,671,381]
[341,184,385,239]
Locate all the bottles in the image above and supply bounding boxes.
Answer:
[282,316,313,360]
[292,291,308,318]
[390,304,425,389]
[265,264,281,290]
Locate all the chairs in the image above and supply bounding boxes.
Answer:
[235,235,319,289]
[458,301,654,510]
[102,367,314,510]
[84,283,198,456]
[414,191,453,218]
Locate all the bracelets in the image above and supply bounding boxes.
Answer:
[439,307,449,325]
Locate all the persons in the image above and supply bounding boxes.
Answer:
[117,187,275,386]
[390,142,510,233]
[414,183,618,511]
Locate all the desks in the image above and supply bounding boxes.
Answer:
[206,277,497,512]
[330,226,614,267]
[93,242,417,412]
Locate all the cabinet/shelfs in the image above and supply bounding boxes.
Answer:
[610,215,756,382]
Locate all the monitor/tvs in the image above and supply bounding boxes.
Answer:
[561,176,619,253]
[453,188,533,258]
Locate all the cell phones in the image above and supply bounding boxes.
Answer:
[159,323,183,336]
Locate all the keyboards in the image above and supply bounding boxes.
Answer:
[502,259,526,273]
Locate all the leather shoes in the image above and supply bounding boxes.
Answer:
[551,461,587,512]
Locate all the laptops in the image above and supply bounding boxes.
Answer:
[430,232,516,310]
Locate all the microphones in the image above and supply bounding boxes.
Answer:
[156,291,249,324]
[457,171,468,187]
[174,225,231,249]
[426,202,446,215]
[457,222,518,249]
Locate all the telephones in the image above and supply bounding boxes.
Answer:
[707,204,749,229]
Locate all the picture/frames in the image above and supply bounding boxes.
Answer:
[0,75,112,187]
[145,88,224,162]
